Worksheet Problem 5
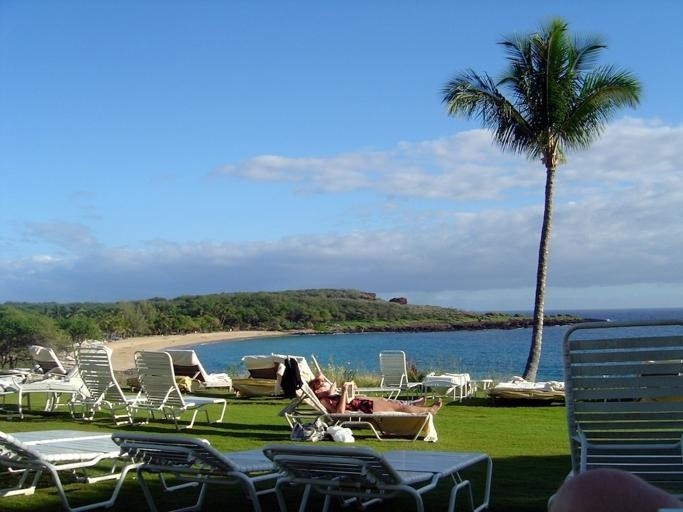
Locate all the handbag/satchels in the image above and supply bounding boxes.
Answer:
[289,421,329,442]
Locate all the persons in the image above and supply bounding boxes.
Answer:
[308,374,442,417]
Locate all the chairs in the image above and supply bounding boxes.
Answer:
[562,322,681,512]
